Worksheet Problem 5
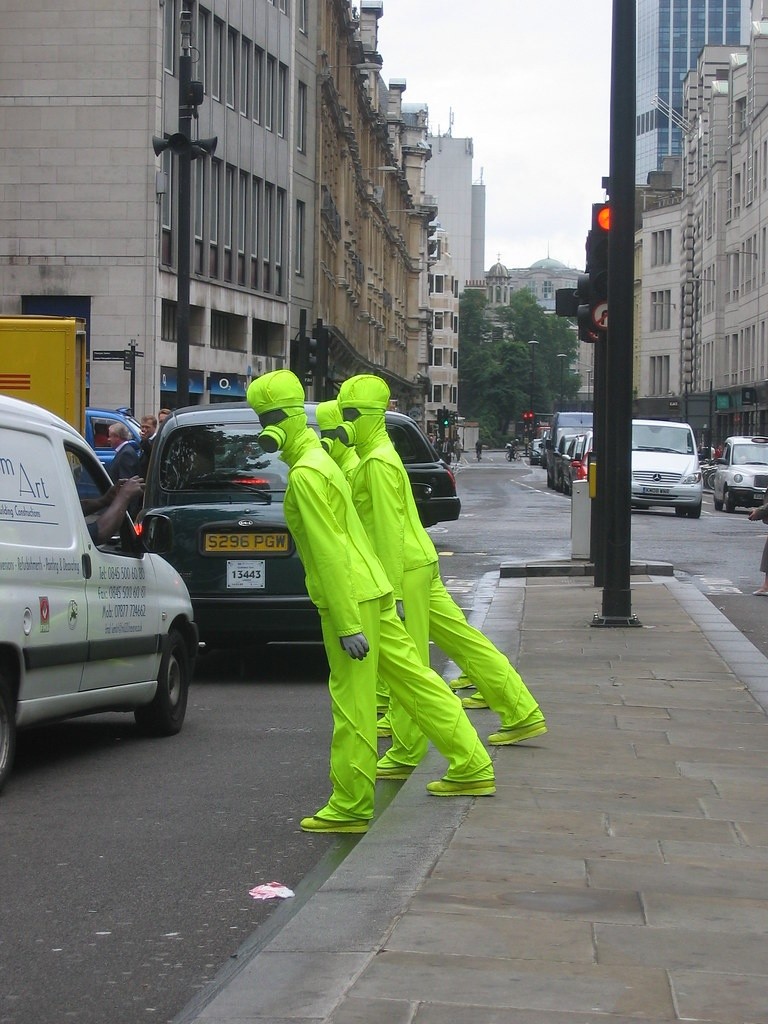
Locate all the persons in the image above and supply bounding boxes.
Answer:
[139,415,157,451]
[748,487,768,596]
[158,409,171,427]
[475,436,483,459]
[697,443,702,454]
[316,400,474,713]
[96,422,145,517]
[508,438,517,462]
[336,375,547,779]
[247,369,497,833]
[429,435,434,444]
[715,444,723,458]
[436,434,463,466]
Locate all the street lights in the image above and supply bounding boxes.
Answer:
[556,354,570,414]
[586,369,591,413]
[178,9,205,401]
[525,339,538,458]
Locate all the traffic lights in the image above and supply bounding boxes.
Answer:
[555,286,582,319]
[583,202,612,301]
[522,413,535,419]
[304,336,322,388]
[576,274,602,343]
[444,409,451,427]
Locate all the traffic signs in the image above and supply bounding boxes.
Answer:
[93,350,131,361]
[134,351,145,357]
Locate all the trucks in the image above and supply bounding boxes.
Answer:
[0,314,88,478]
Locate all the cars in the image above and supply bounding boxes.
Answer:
[383,410,462,528]
[528,411,594,496]
[1,395,201,795]
[712,436,768,512]
[125,399,395,651]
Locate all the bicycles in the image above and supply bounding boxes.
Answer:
[505,445,523,463]
[476,450,482,462]
[698,453,720,491]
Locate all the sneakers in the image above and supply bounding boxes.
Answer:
[449,677,473,689]
[376,766,415,779]
[462,697,488,709]
[300,816,369,834]
[426,778,496,796]
[377,727,392,738]
[377,706,388,713]
[487,720,548,745]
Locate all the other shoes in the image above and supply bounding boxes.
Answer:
[752,591,768,596]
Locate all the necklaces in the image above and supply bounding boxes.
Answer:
[79,474,145,546]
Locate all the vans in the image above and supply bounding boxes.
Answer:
[629,419,712,518]
[74,406,158,540]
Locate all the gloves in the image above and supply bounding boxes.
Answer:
[340,632,369,661]
[396,600,405,621]
